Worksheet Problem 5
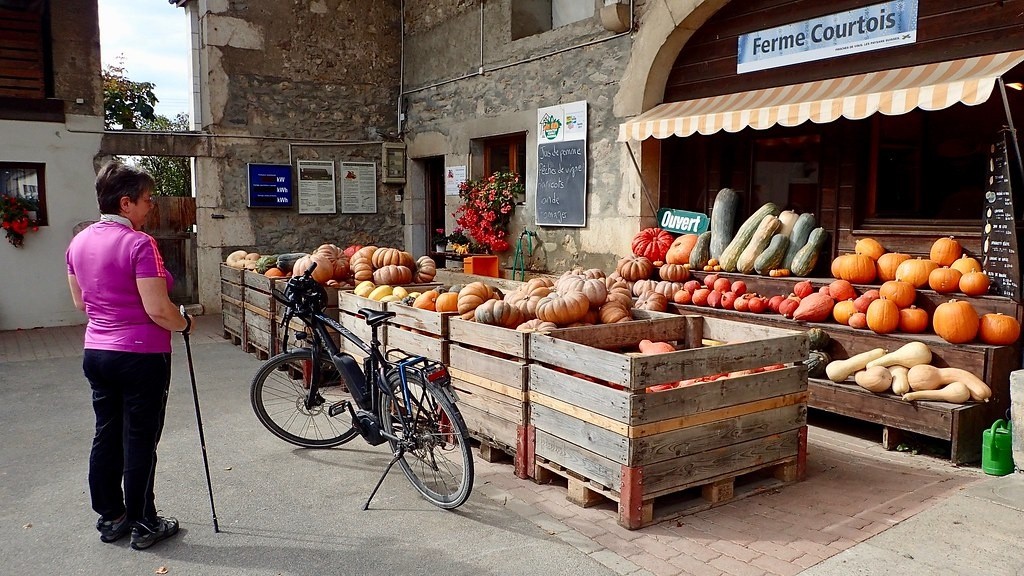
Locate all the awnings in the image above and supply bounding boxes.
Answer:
[614,49,1024,142]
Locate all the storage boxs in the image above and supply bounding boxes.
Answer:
[527,315,810,528]
[338,285,459,398]
[463,256,499,278]
[243,269,288,360]
[446,310,685,481]
[219,262,253,351]
[386,302,459,440]
[275,278,450,389]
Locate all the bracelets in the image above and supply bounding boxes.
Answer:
[176,311,191,333]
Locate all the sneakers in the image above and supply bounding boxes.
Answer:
[130,516,179,550]
[96,507,129,543]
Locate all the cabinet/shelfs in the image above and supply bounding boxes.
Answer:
[653,266,1024,466]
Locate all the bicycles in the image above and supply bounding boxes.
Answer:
[249,263,475,512]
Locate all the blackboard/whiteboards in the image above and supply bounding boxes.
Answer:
[535,139,586,225]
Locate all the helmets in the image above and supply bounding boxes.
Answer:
[288,278,329,316]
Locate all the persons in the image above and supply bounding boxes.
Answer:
[64,161,195,552]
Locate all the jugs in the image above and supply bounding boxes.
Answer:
[982,419,1013,476]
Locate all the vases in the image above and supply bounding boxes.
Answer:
[435,244,446,252]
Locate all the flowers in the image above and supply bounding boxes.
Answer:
[0,194,40,247]
[432,171,525,254]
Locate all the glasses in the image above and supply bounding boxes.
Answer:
[143,196,153,202]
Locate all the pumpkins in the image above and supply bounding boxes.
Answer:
[227,188,1019,403]
